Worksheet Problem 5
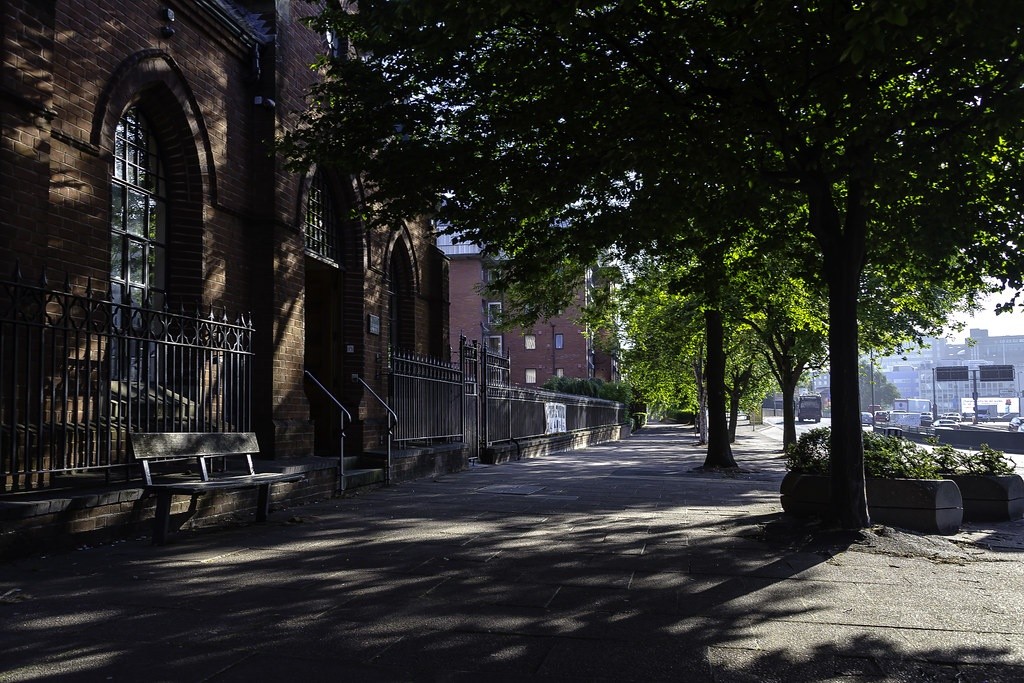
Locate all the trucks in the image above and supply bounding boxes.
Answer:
[975,404,998,420]
[894,398,931,412]
[798,393,822,422]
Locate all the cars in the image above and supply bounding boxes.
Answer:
[1009,417,1024,432]
[860,410,1019,432]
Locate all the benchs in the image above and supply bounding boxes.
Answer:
[127,432,302,544]
